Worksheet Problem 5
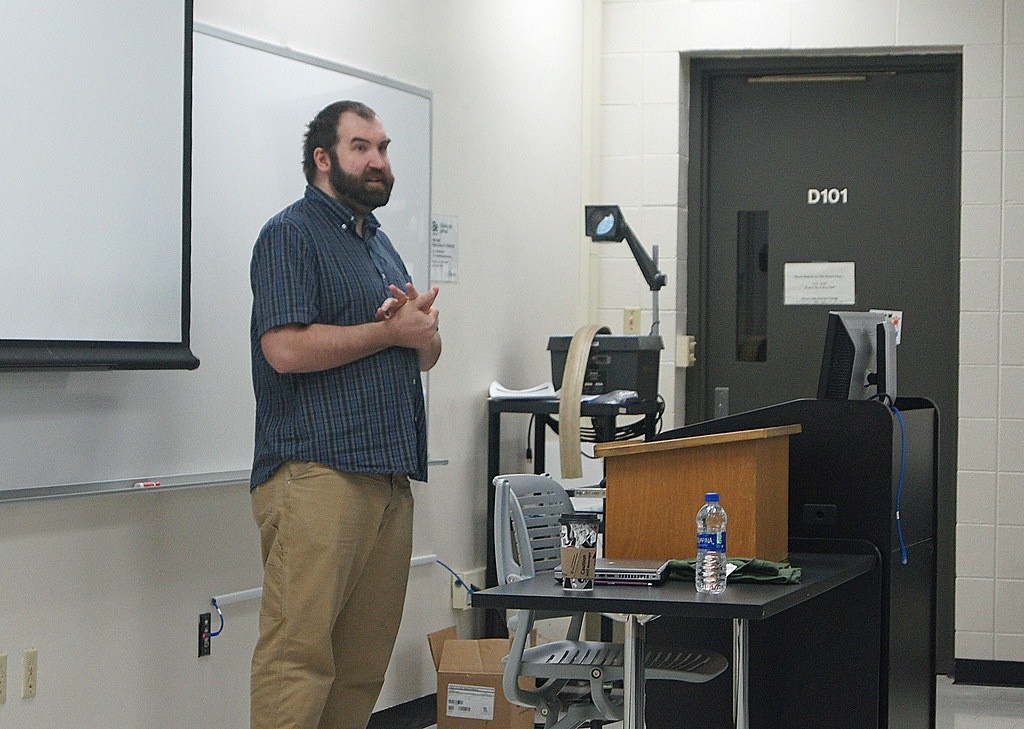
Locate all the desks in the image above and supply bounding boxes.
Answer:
[469,547,885,729]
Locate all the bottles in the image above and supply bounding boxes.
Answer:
[695,493,727,594]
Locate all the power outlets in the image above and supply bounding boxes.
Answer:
[0,654,7,704]
[22,649,37,699]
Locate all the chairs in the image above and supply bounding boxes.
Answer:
[488,474,730,729]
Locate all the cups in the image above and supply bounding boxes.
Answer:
[558,513,600,592]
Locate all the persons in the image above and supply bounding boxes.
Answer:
[250,101,442,729]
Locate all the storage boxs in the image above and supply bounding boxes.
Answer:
[426,625,536,729]
